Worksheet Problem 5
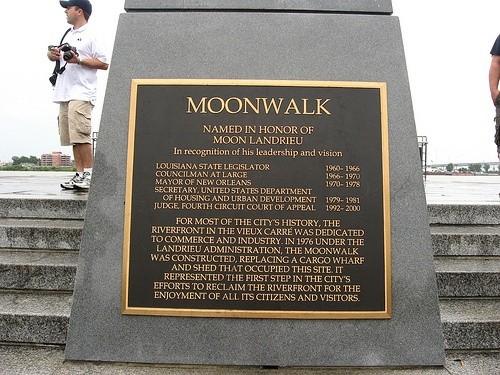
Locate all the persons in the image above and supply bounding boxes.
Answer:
[489,34,500,199]
[46,0,110,191]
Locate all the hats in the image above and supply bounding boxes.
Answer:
[59,0,92,15]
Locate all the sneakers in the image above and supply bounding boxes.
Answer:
[60,173,83,189]
[73,171,92,189]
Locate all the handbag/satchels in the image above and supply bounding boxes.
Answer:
[48,60,61,85]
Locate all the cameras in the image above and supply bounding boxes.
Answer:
[58,43,76,62]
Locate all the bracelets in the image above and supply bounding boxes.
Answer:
[78,58,84,66]
